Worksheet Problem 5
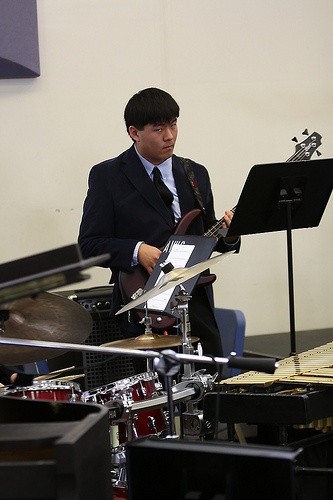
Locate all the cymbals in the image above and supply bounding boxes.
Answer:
[32,366,85,382]
[98,334,200,350]
[0,291,94,366]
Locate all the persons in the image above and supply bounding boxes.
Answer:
[78,87,241,440]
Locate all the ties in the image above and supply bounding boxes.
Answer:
[152,166,176,229]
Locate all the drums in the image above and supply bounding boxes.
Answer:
[81,371,169,440]
[0,379,81,403]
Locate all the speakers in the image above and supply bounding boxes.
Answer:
[47,285,147,392]
[0,0,40,79]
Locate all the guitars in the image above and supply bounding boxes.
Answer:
[118,128,322,329]
